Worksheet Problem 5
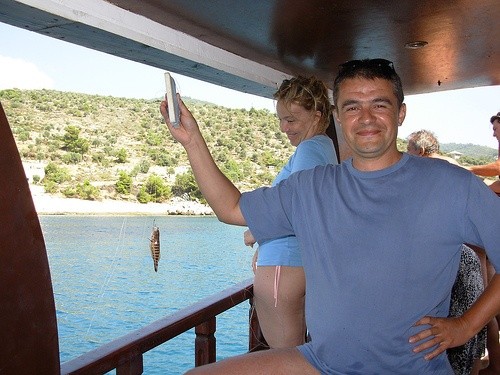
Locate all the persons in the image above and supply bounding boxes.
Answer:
[408,130,500,375]
[242,75,338,349]
[160,58,500,375]
[459,113,499,194]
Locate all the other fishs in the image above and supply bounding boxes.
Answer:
[147,226,161,272]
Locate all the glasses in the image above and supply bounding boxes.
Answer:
[337,58,395,71]
